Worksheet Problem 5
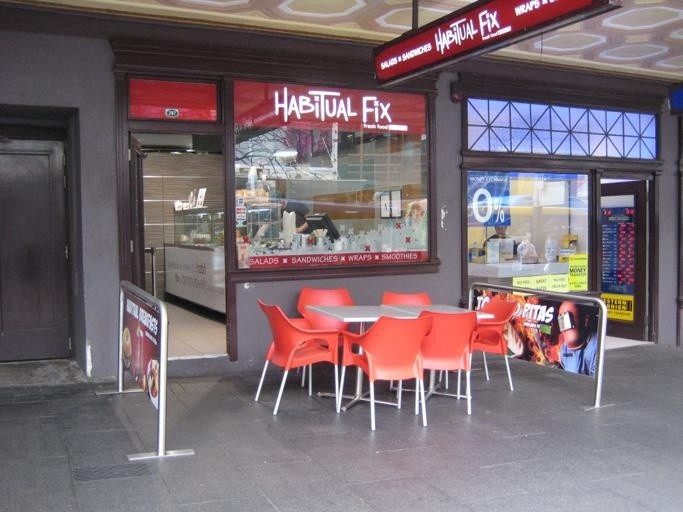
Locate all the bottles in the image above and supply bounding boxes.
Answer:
[471,241,479,258]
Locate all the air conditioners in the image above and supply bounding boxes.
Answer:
[135,131,194,154]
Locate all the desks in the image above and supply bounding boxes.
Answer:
[308,302,496,412]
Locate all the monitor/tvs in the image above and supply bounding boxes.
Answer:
[490,238,514,262]
[305,212,340,242]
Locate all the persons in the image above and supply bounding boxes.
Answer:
[259,190,311,237]
[542,301,598,377]
[480,224,517,256]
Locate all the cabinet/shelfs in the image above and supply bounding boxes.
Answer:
[172,208,230,247]
[242,202,282,242]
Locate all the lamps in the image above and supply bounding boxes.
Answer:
[273,139,298,160]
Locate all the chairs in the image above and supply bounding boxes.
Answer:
[340,313,434,431]
[395,309,480,418]
[438,294,519,393]
[379,289,433,389]
[253,297,339,416]
[295,284,362,398]
